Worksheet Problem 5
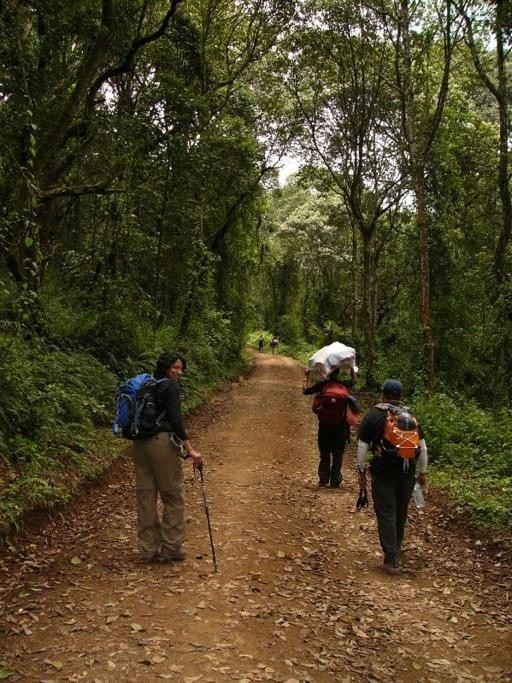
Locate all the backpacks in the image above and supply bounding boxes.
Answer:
[376,403,420,460]
[114,372,171,440]
[312,381,348,421]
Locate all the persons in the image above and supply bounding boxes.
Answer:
[258,335,264,353]
[134,350,204,562]
[357,378,429,576]
[303,365,357,453]
[270,336,278,354]
[311,373,363,489]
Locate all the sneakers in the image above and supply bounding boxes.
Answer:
[160,545,185,559]
[382,562,401,575]
[318,479,340,488]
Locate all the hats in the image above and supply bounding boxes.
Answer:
[381,380,403,396]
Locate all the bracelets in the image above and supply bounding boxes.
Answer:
[304,379,308,381]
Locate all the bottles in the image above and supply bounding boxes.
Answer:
[412,475,426,509]
